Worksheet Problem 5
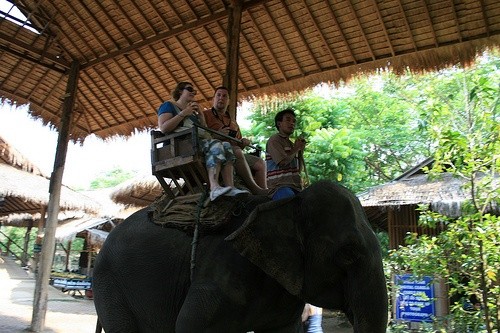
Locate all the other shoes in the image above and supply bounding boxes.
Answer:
[225,186,249,197]
[209,186,232,202]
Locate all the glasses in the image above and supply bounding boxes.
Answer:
[181,87,197,92]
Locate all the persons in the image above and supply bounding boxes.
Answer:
[202,86,271,194]
[157,81,251,200]
[265,107,307,201]
[301,303,323,333]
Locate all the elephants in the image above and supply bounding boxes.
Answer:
[92,181,388,333]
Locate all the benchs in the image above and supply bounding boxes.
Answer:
[150,129,261,198]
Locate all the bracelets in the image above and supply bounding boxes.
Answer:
[180,111,185,116]
[177,113,185,118]
